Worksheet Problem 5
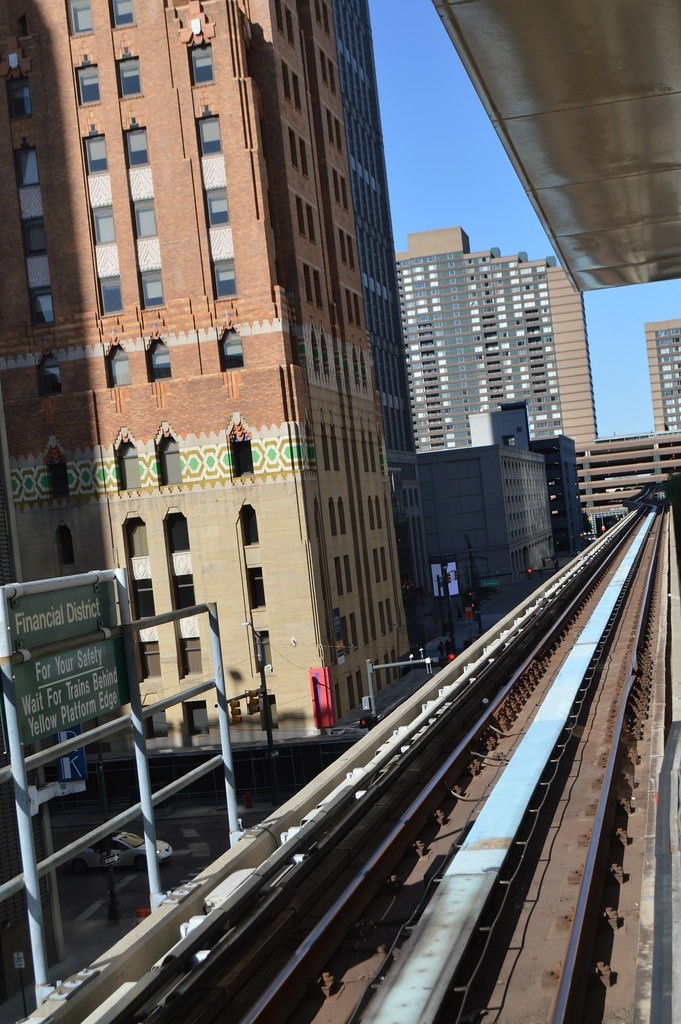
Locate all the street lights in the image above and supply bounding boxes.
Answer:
[442,561,456,651]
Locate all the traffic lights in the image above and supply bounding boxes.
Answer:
[446,653,456,662]
[230,699,243,725]
[246,688,261,715]
[471,603,476,608]
[358,715,369,728]
[528,568,533,574]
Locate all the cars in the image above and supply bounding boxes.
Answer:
[53,830,174,873]
